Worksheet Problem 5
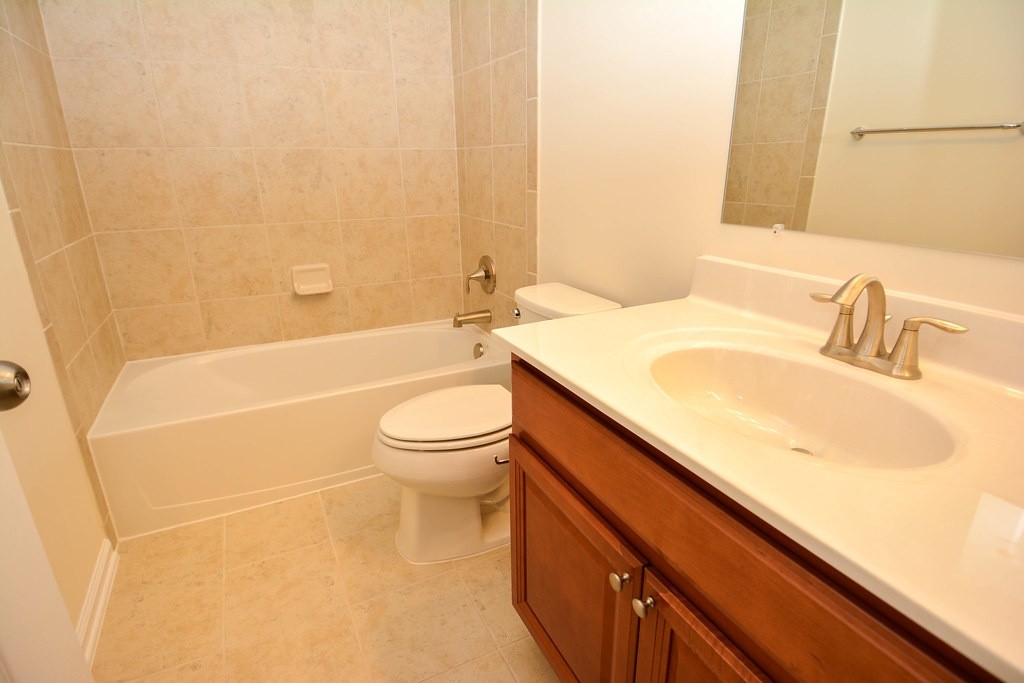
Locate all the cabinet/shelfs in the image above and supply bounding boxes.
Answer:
[508,352,1006,683]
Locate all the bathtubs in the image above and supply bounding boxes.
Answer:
[85,312,512,541]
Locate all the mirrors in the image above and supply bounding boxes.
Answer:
[721,1,1024,261]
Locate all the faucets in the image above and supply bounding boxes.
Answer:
[451,310,492,328]
[828,270,895,367]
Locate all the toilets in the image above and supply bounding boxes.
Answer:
[368,277,628,566]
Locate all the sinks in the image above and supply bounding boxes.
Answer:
[628,325,970,494]
